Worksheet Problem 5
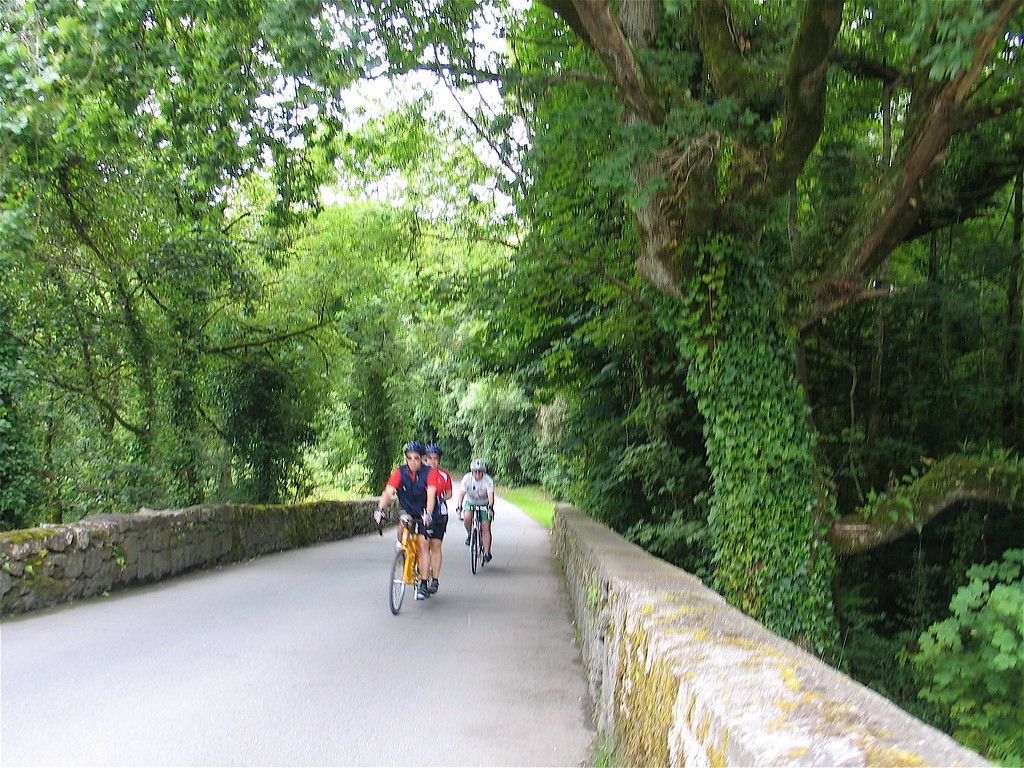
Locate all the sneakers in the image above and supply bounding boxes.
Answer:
[427,579,439,594]
[415,586,429,600]
[464,535,472,545]
[483,552,494,565]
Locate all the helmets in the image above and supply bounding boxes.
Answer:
[471,459,487,472]
[422,444,444,457]
[402,440,428,455]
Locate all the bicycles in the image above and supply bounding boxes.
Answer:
[378,515,436,617]
[457,504,494,575]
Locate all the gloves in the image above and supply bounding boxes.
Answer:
[374,507,387,524]
[419,511,434,528]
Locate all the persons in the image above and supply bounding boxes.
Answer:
[421,444,452,594]
[456,460,496,562]
[372,442,436,600]
